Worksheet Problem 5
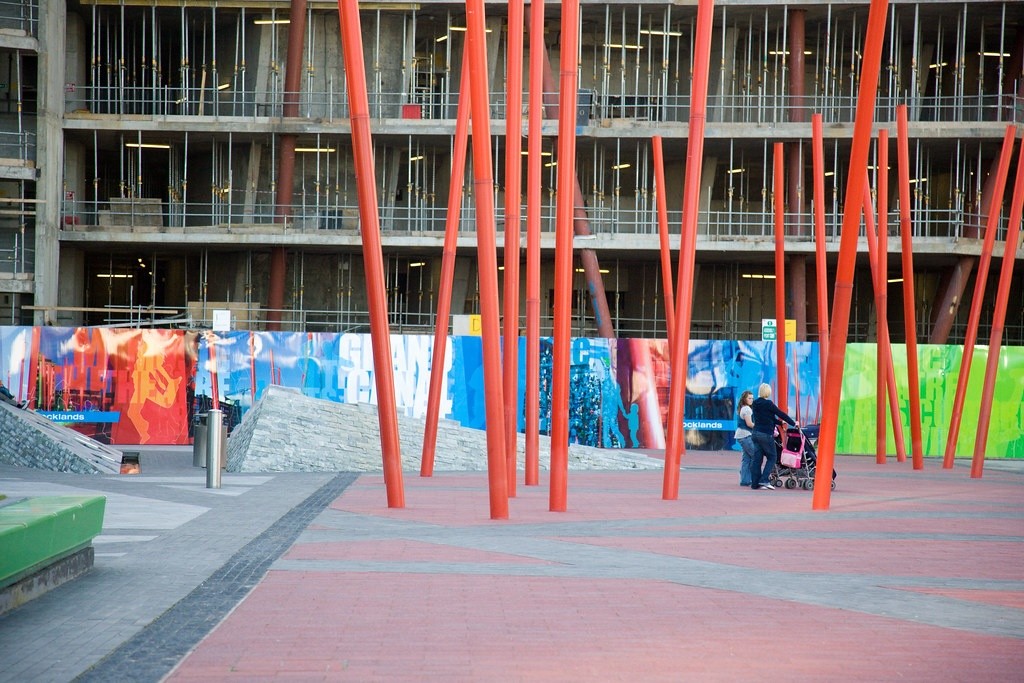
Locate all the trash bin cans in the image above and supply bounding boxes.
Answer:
[192,413,229,468]
[575,86,593,126]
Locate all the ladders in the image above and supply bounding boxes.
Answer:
[410,53,434,120]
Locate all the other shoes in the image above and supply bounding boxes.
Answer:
[759,482,775,490]
[755,485,769,490]
[740,481,752,486]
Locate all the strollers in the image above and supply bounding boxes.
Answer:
[774,421,837,492]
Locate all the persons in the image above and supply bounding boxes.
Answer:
[734,390,754,486]
[749,383,800,490]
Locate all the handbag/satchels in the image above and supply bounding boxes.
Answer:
[780,448,802,468]
